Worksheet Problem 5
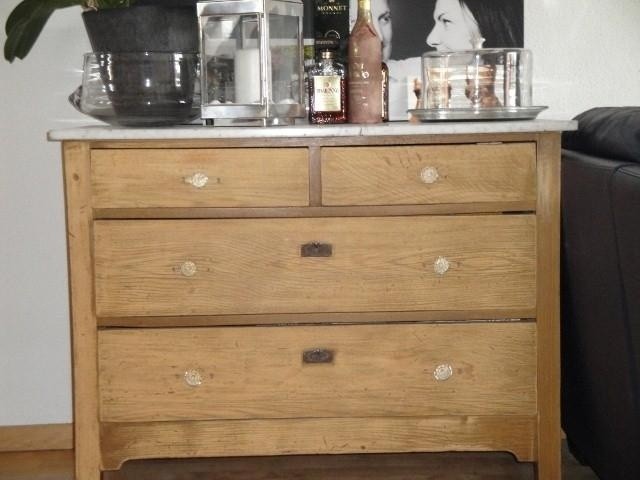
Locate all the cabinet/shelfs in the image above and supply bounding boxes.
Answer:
[62,131,563,479]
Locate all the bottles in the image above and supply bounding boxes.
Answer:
[308,0,383,125]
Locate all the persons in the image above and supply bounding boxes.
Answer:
[426,1,524,54]
[349,1,393,63]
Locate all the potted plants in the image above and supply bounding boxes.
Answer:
[3,0,201,128]
